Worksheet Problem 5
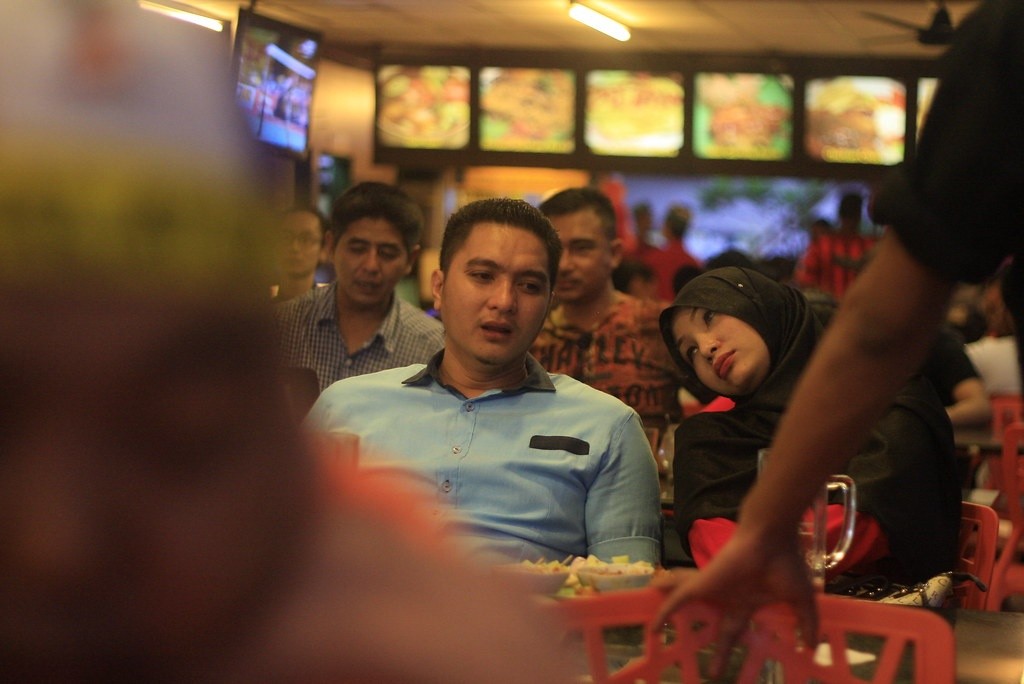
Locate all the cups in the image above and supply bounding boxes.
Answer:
[758,448,856,586]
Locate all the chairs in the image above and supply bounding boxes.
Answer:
[548,393,1023,684]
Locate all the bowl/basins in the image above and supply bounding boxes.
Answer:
[491,564,570,595]
[576,564,655,592]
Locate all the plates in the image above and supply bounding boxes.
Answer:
[792,642,877,674]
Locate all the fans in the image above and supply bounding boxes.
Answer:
[851,0,957,46]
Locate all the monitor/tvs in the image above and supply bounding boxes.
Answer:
[233,7,321,159]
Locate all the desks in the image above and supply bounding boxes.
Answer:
[953,426,1024,455]
[577,601,1023,684]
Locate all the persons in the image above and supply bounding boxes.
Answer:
[526,186,674,428]
[658,266,964,618]
[645,207,702,302]
[814,191,877,293]
[294,196,662,569]
[622,201,660,260]
[794,218,834,286]
[0,124,580,684]
[268,204,328,306]
[276,177,446,392]
[928,321,994,427]
[651,0,1023,684]
